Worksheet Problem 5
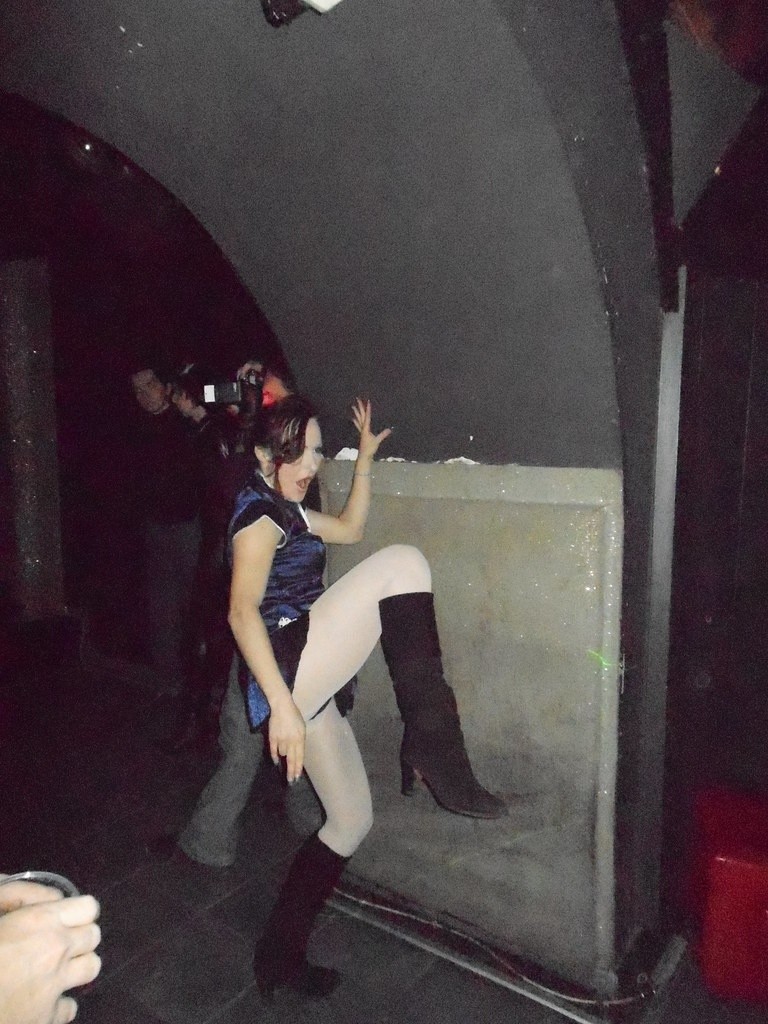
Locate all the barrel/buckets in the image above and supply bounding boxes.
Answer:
[680,771,767,1009]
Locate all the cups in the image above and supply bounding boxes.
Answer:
[1,869,83,925]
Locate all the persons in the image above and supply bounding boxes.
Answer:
[228,396,504,1003]
[127,365,204,701]
[163,372,245,758]
[165,361,322,868]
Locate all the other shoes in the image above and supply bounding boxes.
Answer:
[136,828,199,876]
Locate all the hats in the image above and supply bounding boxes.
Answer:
[172,362,210,398]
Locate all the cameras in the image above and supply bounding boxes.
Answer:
[201,370,264,423]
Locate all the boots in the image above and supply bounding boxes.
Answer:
[239,840,354,1008]
[375,587,508,823]
